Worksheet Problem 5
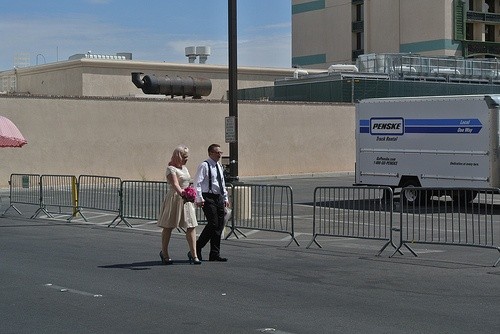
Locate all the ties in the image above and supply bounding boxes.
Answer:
[216,163,224,196]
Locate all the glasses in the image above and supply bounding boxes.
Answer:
[212,151,224,155]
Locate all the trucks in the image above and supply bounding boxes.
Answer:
[353,93,500,206]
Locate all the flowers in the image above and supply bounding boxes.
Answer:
[181,185,196,204]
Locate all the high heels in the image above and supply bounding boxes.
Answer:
[160,251,174,265]
[187,252,202,264]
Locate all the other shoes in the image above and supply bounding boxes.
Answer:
[196,241,202,260]
[209,256,228,261]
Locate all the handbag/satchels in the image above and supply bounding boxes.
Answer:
[222,206,232,231]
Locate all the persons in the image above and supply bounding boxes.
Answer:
[193,144,230,262]
[157,146,204,265]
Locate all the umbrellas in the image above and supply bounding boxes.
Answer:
[0,115,27,148]
